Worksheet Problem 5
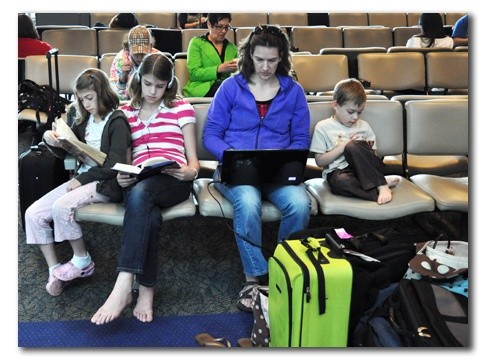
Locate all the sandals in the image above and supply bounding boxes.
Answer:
[52,260,95,281]
[237,279,261,312]
[46,274,72,296]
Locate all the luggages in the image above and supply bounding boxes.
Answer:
[268,233,354,347]
[18,141,70,244]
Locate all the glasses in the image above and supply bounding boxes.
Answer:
[249,25,284,42]
[214,24,231,30]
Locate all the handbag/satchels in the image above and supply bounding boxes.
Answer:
[367,278,467,347]
[18,79,72,118]
[344,226,415,297]
[402,237,468,299]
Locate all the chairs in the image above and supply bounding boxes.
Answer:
[177,13,268,30]
[382,94,469,173]
[19,55,100,130]
[428,51,468,88]
[454,46,468,52]
[305,94,402,173]
[181,97,220,170]
[343,27,394,48]
[173,53,191,96]
[98,29,182,59]
[40,29,98,56]
[34,13,92,29]
[407,13,445,27]
[100,52,174,81]
[393,26,453,47]
[328,13,368,27]
[403,98,470,212]
[290,55,348,92]
[380,46,454,52]
[268,13,329,29]
[72,103,197,225]
[317,46,386,98]
[234,27,291,53]
[290,54,349,100]
[182,28,235,55]
[287,52,313,55]
[292,27,343,53]
[368,13,407,27]
[305,100,436,221]
[445,14,468,26]
[91,13,177,34]
[426,52,469,92]
[193,103,319,220]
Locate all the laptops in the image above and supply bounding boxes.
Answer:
[217,149,309,187]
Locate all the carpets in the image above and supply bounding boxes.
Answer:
[19,314,269,347]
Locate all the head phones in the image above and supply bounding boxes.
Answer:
[135,51,174,89]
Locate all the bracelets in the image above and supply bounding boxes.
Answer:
[190,167,198,180]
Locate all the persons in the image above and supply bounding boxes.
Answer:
[202,24,313,313]
[406,13,454,50]
[89,54,200,327]
[310,79,401,205]
[18,15,54,58]
[107,25,163,101]
[178,13,208,29]
[26,68,131,297]
[451,13,468,45]
[182,13,242,97]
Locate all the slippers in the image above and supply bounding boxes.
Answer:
[195,333,232,347]
[237,337,257,347]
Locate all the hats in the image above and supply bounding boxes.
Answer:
[128,25,153,55]
